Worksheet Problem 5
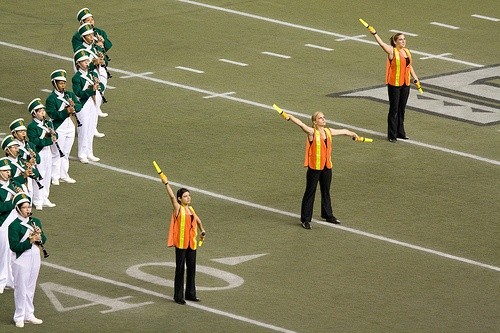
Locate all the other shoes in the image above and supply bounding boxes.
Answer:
[99,112,108,118]
[80,155,100,164]
[16,321,24,327]
[36,203,56,210]
[326,216,340,224]
[301,221,312,230]
[95,130,105,137]
[195,298,200,302]
[174,298,186,305]
[51,177,76,185]
[24,317,43,325]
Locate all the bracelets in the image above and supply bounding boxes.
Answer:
[164,182,168,185]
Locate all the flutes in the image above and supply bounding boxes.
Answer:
[63,87,83,127]
[8,174,21,194]
[23,136,43,180]
[42,115,65,158]
[91,24,111,62]
[87,64,108,104]
[26,212,49,258]
[16,151,44,190]
[93,42,113,79]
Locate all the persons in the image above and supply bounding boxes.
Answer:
[0,7,112,294]
[285,112,360,229]
[161,176,206,305]
[371,28,420,143]
[8,199,47,327]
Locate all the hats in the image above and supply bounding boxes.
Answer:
[77,7,93,22]
[28,98,46,116]
[9,117,27,132]
[12,193,31,208]
[78,23,94,38]
[1,135,20,152]
[0,157,12,170]
[50,69,67,82]
[74,49,90,64]
